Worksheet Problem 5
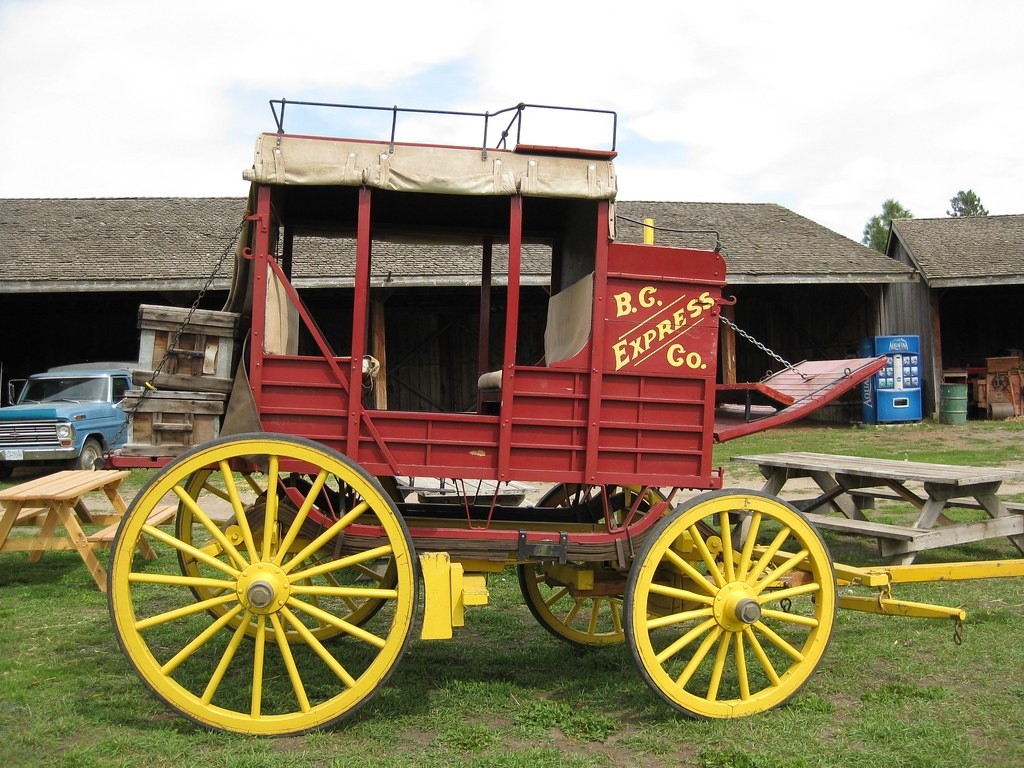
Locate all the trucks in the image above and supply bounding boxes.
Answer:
[0,362,139,480]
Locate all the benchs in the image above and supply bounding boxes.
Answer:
[731,510,944,543]
[87,505,178,542]
[478,271,594,388]
[0,508,50,524]
[847,488,1024,513]
[264,262,380,379]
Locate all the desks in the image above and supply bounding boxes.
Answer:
[0,470,157,593]
[705,451,1024,576]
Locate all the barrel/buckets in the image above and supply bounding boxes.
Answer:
[939,382,969,425]
[988,402,1015,421]
